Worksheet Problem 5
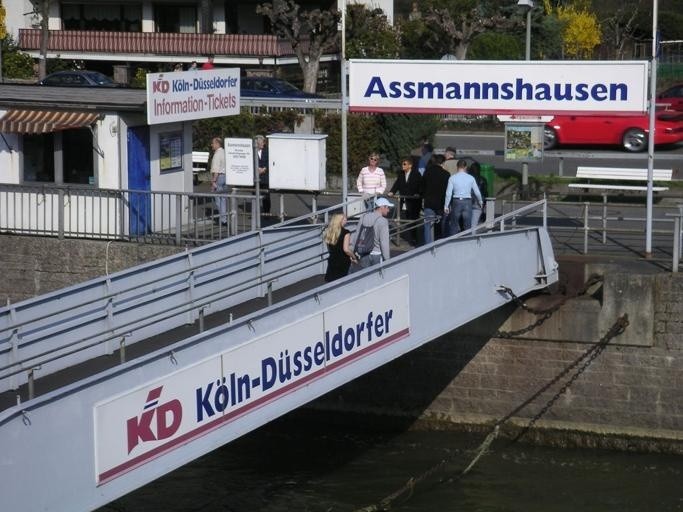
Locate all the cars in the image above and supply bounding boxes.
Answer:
[190,152,209,174]
[32,67,131,88]
[239,73,327,114]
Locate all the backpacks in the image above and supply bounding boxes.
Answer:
[354,214,381,256]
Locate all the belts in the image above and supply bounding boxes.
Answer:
[454,198,472,200]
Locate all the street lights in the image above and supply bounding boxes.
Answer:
[516,0,535,62]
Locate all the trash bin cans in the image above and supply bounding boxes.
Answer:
[480,163,495,198]
[113,65,128,83]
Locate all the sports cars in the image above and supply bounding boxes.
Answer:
[542,93,683,155]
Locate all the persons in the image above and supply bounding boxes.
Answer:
[174,52,215,71]
[350,198,395,270]
[423,146,487,245]
[356,152,388,212]
[210,137,228,226]
[388,158,424,247]
[324,213,356,283]
[252,134,271,220]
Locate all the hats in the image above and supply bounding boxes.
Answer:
[375,197,395,207]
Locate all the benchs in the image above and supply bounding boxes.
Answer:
[565,164,673,204]
[193,148,212,176]
